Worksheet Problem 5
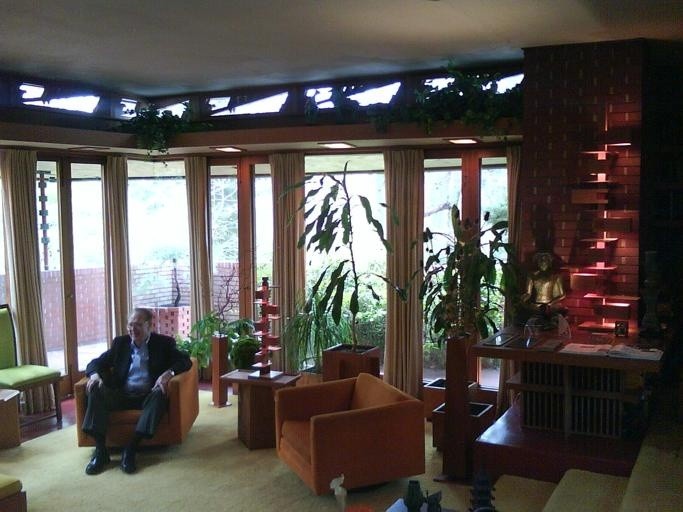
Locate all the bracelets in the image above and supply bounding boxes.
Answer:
[167,369,175,376]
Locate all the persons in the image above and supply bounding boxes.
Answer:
[521,249,568,314]
[85,306,193,477]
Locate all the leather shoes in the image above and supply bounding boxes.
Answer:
[85,451,112,475]
[119,446,137,474]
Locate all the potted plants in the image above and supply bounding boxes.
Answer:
[409,209,520,475]
[284,286,347,385]
[165,307,241,388]
[282,160,404,379]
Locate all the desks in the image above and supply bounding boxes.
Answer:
[1,386,24,451]
[217,364,302,452]
[469,322,667,443]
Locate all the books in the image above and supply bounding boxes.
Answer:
[536,338,663,360]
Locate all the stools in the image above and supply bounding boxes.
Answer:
[1,472,26,512]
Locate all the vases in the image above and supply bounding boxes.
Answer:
[423,378,478,421]
[432,399,495,448]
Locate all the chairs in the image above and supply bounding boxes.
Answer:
[1,302,64,432]
[269,370,428,499]
[72,355,201,451]
